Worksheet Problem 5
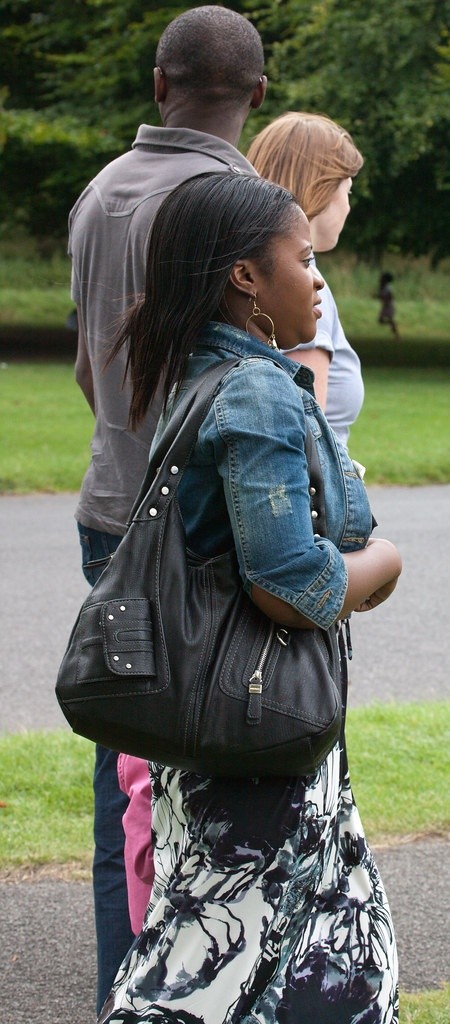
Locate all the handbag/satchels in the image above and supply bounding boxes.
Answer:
[54,355,341,782]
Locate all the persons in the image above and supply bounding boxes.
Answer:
[373,270,400,338]
[98,172,403,1024]
[245,111,365,448]
[67,6,269,1013]
